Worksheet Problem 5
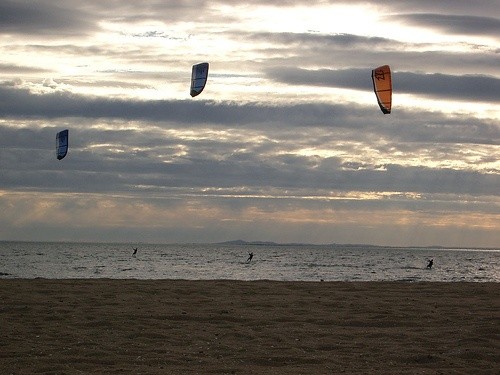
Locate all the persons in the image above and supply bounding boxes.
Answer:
[247,252,253,262]
[427,259,433,269]
[133,247,137,255]
